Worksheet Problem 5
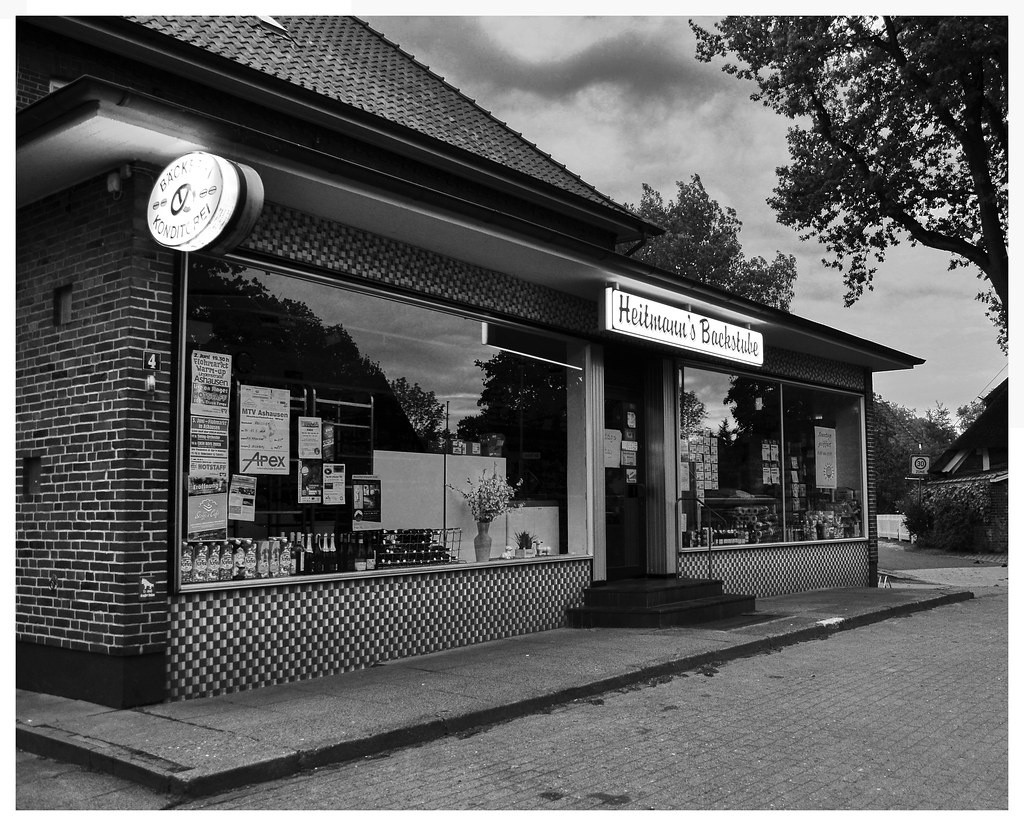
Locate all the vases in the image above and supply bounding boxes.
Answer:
[474,523,492,562]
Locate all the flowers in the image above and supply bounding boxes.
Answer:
[445,461,527,522]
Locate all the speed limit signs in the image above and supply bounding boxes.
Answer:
[915,458,926,469]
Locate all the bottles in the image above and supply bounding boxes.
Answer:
[280,532,314,575]
[338,531,378,571]
[314,533,338,573]
[713,521,749,545]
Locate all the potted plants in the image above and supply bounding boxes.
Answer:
[508,529,537,558]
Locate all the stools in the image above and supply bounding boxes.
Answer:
[876,570,896,588]
